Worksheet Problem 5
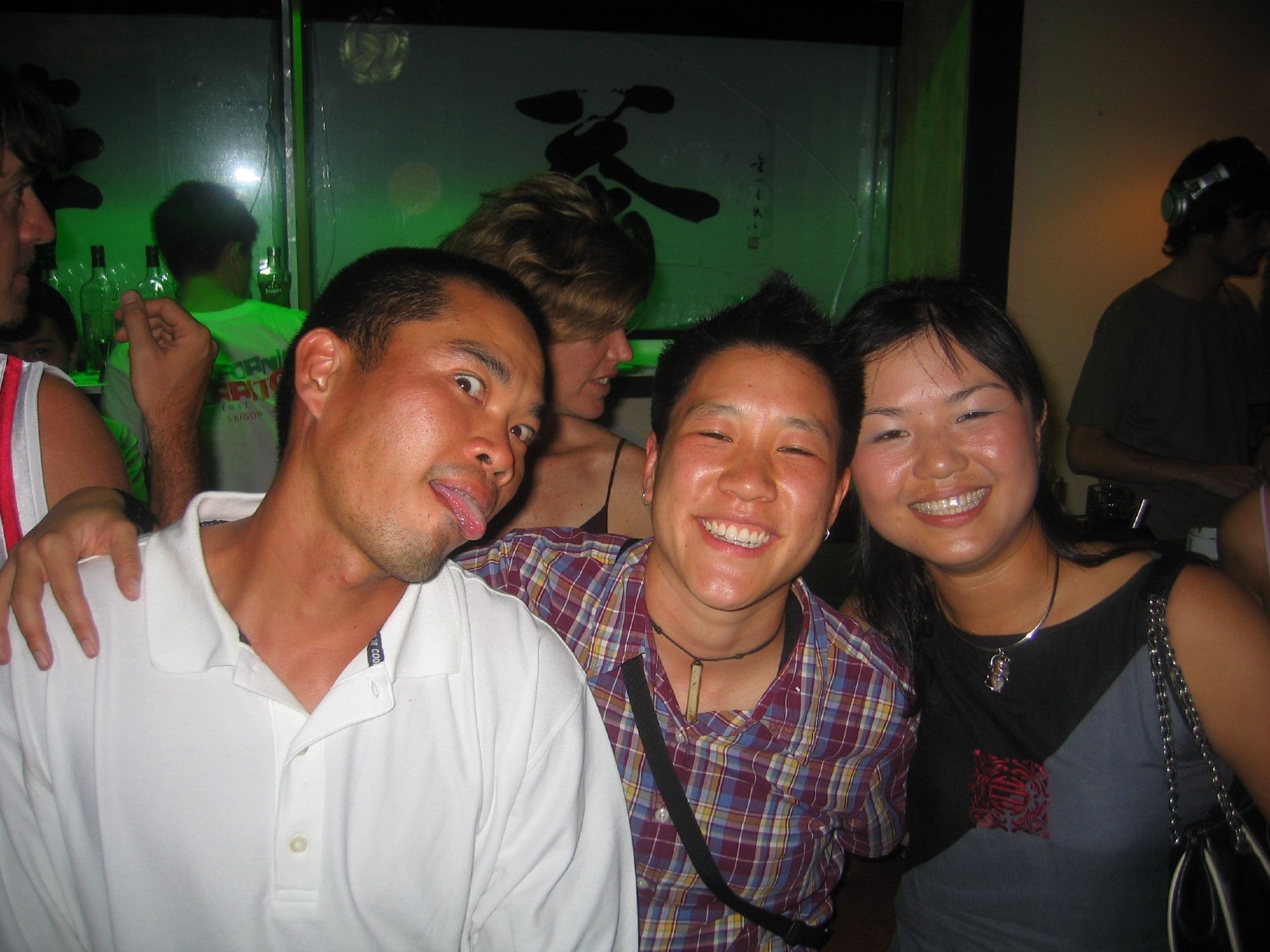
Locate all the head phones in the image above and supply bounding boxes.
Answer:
[1162,162,1233,223]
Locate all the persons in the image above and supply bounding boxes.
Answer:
[439,180,655,538]
[838,279,1270,952]
[0,267,922,952]
[0,246,638,952]
[0,89,310,566]
[1067,136,1270,612]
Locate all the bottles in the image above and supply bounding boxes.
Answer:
[80,244,120,376]
[254,246,292,310]
[137,244,175,304]
[45,263,72,302]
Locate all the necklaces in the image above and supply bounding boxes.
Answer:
[934,546,1059,693]
[648,615,784,725]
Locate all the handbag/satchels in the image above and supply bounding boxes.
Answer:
[1144,547,1270,952]
